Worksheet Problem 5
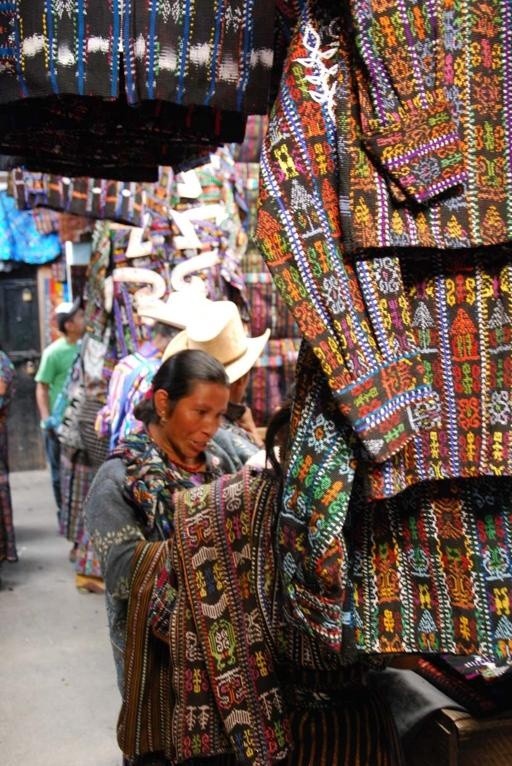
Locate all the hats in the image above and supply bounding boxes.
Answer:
[161,300,272,385]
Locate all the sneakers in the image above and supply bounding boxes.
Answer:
[77,574,106,594]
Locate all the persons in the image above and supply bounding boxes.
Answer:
[1,344,19,565]
[34,296,86,509]
[79,344,264,694]
[161,299,272,448]
[75,324,183,593]
